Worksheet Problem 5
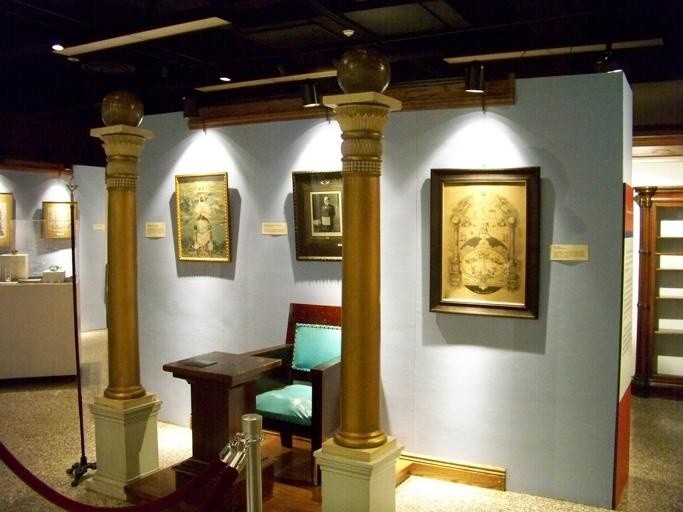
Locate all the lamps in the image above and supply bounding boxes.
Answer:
[183,92,199,118]
[301,77,321,108]
[463,60,486,93]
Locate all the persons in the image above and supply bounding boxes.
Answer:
[316,196,335,233]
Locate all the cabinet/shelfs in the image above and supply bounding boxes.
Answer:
[632,186,683,401]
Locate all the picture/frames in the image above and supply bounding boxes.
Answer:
[429,166,541,320]
[41,201,79,241]
[174,171,231,264]
[291,170,344,263]
[0,193,14,247]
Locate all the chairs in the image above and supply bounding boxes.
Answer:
[239,302,345,489]
